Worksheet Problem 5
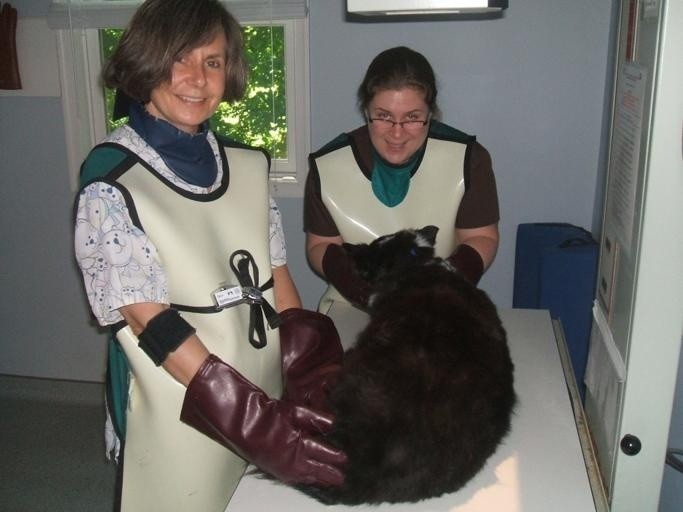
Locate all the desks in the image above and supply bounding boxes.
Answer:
[223,301,597,511]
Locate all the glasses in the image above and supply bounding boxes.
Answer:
[365,104,431,131]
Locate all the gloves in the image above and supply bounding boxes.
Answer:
[276,308,351,425]
[179,351,348,490]
[323,243,486,317]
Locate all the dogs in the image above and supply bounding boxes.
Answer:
[242,225,519,507]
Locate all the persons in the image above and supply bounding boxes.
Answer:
[302,46,501,352]
[71,1,349,512]
